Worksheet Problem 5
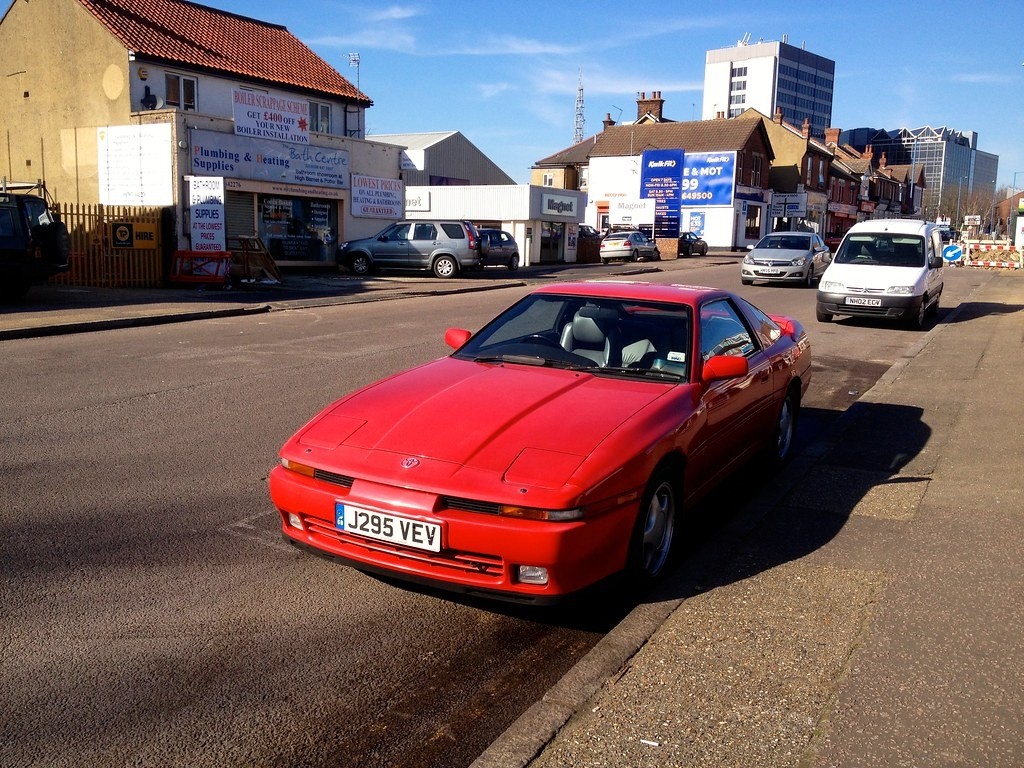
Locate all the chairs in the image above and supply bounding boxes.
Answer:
[645,337,686,379]
[559,311,618,369]
[855,243,877,260]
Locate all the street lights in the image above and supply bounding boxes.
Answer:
[989,180,995,234]
[957,176,968,220]
[909,136,918,209]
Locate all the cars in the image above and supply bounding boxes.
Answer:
[476,229,520,270]
[938,225,960,241]
[578,225,600,238]
[741,232,832,288]
[600,231,660,264]
[678,232,708,256]
[824,231,847,252]
[269,279,812,607]
[816,219,944,328]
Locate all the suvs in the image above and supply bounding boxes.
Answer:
[0,192,71,294]
[335,221,482,278]
